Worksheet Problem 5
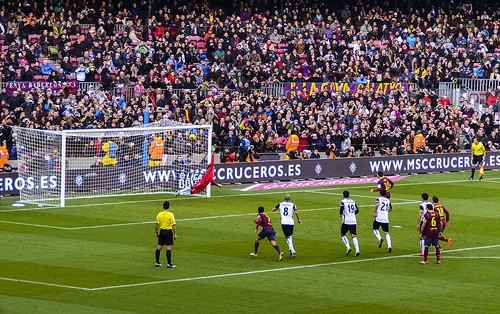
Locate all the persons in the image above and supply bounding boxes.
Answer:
[372,188,392,253]
[232,0,500,89]
[0,0,246,89]
[416,193,435,256]
[272,194,302,259]
[419,204,442,264]
[155,201,177,268]
[0,84,96,172]
[88,86,299,164]
[469,136,486,181]
[340,191,360,257]
[250,206,284,261]
[174,152,222,198]
[432,196,452,253]
[284,84,500,159]
[370,171,393,212]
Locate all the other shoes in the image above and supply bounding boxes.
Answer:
[155,263,162,267]
[420,260,428,265]
[354,251,360,257]
[377,238,384,248]
[387,247,392,253]
[167,263,176,268]
[346,248,352,255]
[292,251,297,258]
[437,260,441,264]
[250,252,258,257]
[278,252,284,262]
[288,248,292,255]
[447,239,452,248]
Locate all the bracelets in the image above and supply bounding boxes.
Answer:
[156,231,158,234]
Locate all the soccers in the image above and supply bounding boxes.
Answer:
[188,134,197,141]
